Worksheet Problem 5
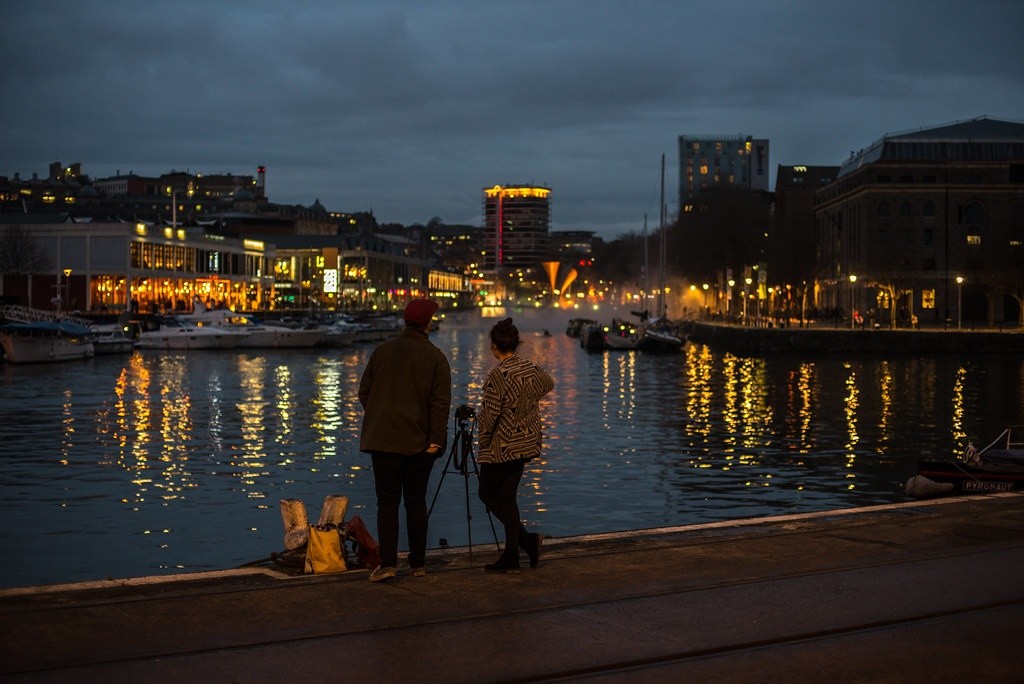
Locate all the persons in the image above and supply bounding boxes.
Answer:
[357,297,451,580]
[475,318,556,572]
[911,313,920,330]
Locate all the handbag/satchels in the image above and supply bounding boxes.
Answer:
[347,516,382,572]
[303,522,347,574]
[338,526,365,569]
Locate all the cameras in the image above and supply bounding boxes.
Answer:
[456,405,475,419]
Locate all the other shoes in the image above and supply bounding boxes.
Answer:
[408,567,425,577]
[368,565,396,581]
[524,531,543,568]
[484,553,521,574]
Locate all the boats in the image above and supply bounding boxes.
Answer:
[0,309,443,364]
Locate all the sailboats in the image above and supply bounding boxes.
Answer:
[565,152,693,355]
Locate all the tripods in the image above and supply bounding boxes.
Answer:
[428,419,502,563]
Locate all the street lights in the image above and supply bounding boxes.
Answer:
[62,265,73,316]
[847,271,858,332]
[954,274,965,330]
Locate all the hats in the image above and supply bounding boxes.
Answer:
[404,299,438,331]
